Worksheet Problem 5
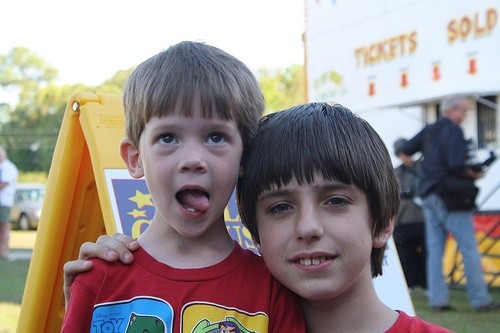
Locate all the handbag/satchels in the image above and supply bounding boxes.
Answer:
[437,177,479,210]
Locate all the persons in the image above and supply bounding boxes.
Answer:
[0,147,20,258]
[389,96,500,312]
[59,42,306,333]
[63,101,455,333]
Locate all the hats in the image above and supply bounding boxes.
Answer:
[393,137,408,155]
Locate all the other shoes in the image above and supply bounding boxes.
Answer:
[440,306,454,312]
[477,301,500,312]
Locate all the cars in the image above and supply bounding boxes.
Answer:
[11,184,46,230]
